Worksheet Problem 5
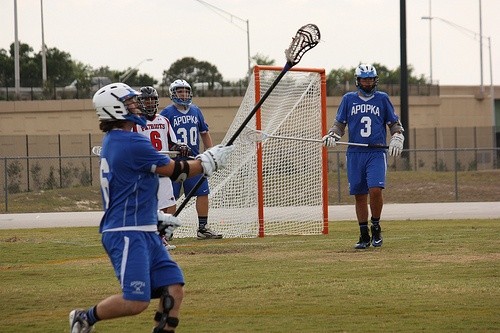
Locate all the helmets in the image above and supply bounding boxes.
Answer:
[169,78,193,106]
[137,86,159,118]
[92,82,147,132]
[354,62,378,97]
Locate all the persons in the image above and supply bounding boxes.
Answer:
[159,79,224,241]
[69,82,235,333]
[322,64,404,249]
[129,86,191,250]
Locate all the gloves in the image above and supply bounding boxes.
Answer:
[387,132,404,158]
[194,143,235,177]
[158,210,182,240]
[322,131,342,147]
[175,142,192,157]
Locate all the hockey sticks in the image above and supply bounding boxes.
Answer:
[245,128,389,149]
[91,145,192,157]
[159,23,322,235]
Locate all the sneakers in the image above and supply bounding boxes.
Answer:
[68,308,94,333]
[197,227,223,239]
[162,239,178,249]
[370,225,383,247]
[355,233,371,248]
